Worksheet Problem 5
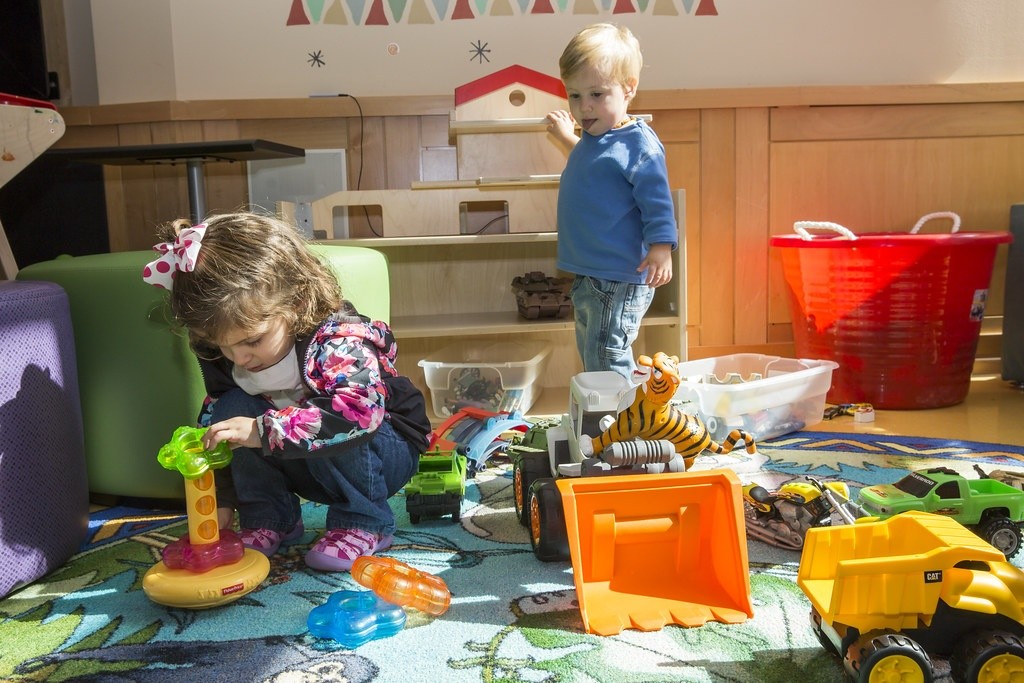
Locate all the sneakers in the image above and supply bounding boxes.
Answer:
[304,528,393,572]
[236,514,304,558]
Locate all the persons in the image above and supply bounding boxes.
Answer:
[143,212,432,571]
[547,24,677,389]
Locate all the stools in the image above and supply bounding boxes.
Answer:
[0,280,90,599]
[21,240,391,512]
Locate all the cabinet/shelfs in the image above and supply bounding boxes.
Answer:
[276,189,688,431]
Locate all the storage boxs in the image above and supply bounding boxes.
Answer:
[675,351,840,446]
[417,338,557,419]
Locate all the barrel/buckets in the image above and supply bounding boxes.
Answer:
[768,208,1011,412]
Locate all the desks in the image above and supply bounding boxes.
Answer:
[42,137,305,228]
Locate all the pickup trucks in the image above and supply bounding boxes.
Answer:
[853,466,1024,563]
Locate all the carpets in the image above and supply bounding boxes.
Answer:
[0,428,1024,683]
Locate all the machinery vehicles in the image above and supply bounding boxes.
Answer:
[403,443,467,524]
[505,370,756,637]
[796,509,1024,683]
